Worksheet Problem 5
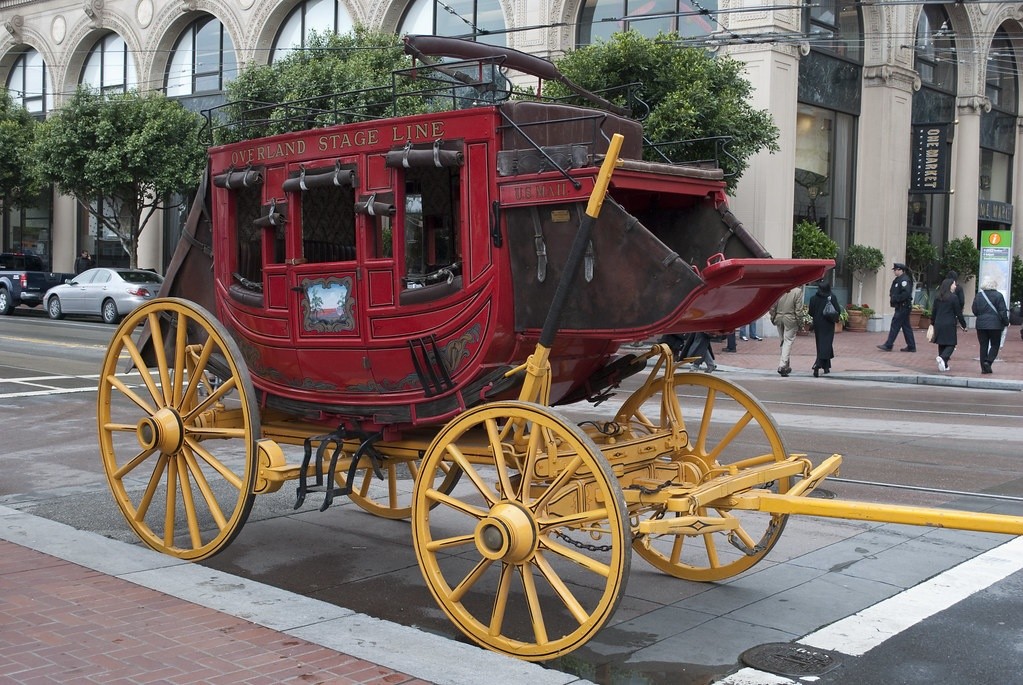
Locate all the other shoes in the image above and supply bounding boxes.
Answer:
[750,335,760,340]
[740,336,748,341]
[981,361,993,374]
[690,364,699,371]
[900,346,916,353]
[704,364,718,373]
[777,366,792,376]
[722,347,736,353]
[935,355,950,371]
[877,344,892,352]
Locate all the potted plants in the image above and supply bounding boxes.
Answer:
[831,306,849,332]
[920,234,982,329]
[907,231,937,328]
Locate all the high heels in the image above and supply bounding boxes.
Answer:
[812,362,819,377]
[824,368,829,374]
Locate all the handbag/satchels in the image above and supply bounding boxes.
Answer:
[927,324,937,344]
[823,294,839,323]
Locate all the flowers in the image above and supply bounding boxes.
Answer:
[844,304,873,314]
[797,305,815,325]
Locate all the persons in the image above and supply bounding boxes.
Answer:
[74,250,95,274]
[770,287,804,377]
[722,332,736,352]
[947,270,965,309]
[972,276,1010,374]
[661,331,718,373]
[808,282,840,378]
[740,321,759,341]
[930,278,967,371]
[877,262,916,352]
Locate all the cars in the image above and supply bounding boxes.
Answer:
[42,267,166,324]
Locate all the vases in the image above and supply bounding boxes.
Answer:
[846,311,868,332]
[799,323,813,336]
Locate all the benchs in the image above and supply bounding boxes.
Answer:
[230,238,355,305]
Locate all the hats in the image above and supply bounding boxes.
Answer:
[891,263,906,271]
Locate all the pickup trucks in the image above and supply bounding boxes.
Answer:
[0,252,77,315]
